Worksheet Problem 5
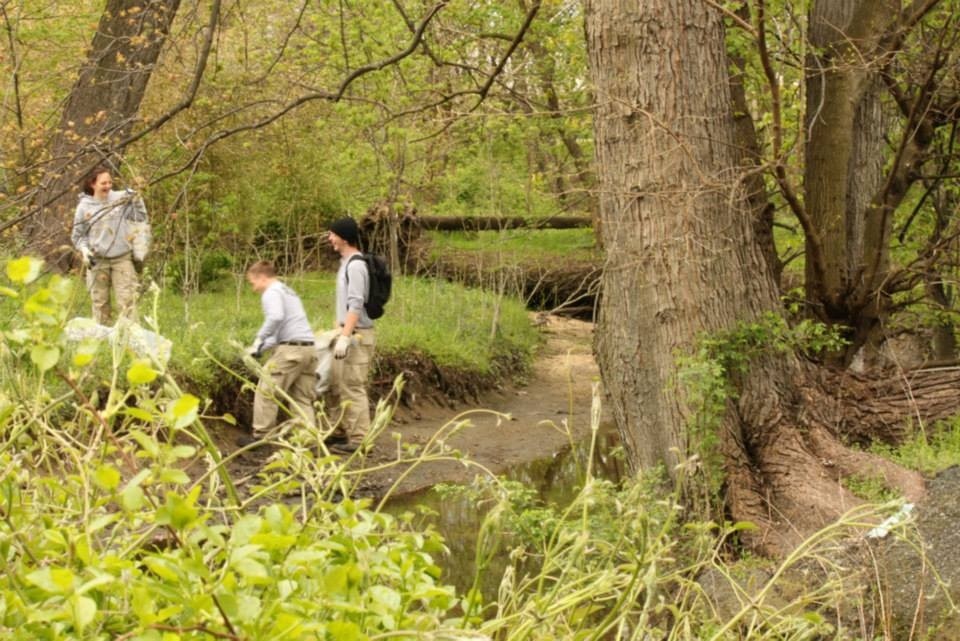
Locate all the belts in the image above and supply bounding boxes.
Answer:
[339,323,372,335]
[279,341,314,347]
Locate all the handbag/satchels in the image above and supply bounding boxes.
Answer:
[124,218,152,261]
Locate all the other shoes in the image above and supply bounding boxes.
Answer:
[322,431,349,446]
[238,434,270,451]
[329,442,373,457]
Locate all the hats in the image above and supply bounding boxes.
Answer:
[327,216,361,245]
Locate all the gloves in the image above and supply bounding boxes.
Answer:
[333,334,349,359]
[251,337,265,356]
[82,247,95,271]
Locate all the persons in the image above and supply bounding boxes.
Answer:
[71,165,145,325]
[234,259,317,457]
[324,216,373,455]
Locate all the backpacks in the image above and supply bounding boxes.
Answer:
[346,253,393,319]
